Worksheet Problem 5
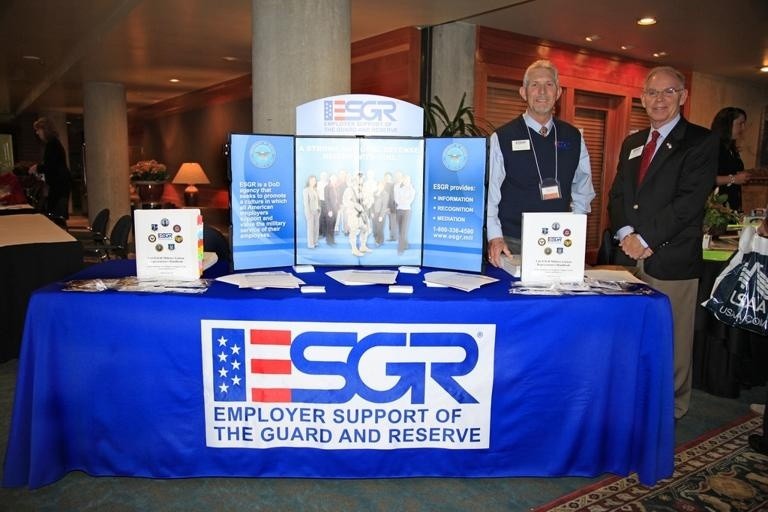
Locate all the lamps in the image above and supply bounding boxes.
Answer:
[170,161,211,209]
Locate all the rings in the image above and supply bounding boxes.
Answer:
[488,257,491,260]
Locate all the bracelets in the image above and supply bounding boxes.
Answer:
[727,174,735,187]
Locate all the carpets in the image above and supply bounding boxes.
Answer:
[525,405,768,512]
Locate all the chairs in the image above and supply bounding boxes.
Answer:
[83,213,134,267]
[66,206,114,264]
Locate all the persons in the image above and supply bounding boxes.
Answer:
[486,59,597,269]
[711,106,752,240]
[747,215,768,456]
[607,65,721,423]
[28,117,71,228]
[302,171,416,257]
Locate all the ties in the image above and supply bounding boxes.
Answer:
[540,127,548,137]
[639,131,659,181]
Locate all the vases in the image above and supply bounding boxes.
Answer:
[136,181,165,209]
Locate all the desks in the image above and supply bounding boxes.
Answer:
[0,263,677,490]
[0,210,81,360]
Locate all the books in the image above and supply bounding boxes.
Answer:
[498,253,521,279]
[202,252,218,272]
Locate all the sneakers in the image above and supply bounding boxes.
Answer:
[750,403,766,415]
[747,432,765,455]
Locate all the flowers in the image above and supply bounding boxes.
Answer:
[128,157,169,182]
